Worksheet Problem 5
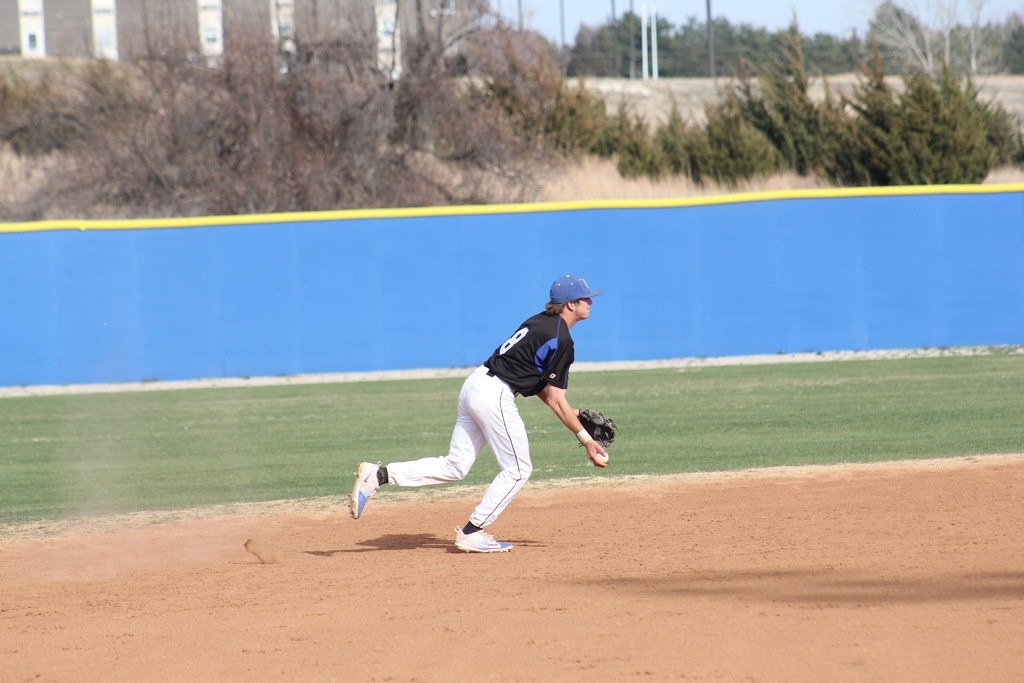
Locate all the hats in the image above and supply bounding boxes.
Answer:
[550,275,602,303]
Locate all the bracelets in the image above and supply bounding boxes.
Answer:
[575,428,592,444]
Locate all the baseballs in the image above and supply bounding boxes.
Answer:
[597,451,609,464]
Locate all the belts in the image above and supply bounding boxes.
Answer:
[486,369,521,399]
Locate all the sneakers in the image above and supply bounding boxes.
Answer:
[347,461,383,520]
[453,526,513,553]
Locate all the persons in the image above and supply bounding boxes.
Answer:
[440,275,446,275]
[347,275,607,552]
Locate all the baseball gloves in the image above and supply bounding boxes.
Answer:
[577,409,616,448]
